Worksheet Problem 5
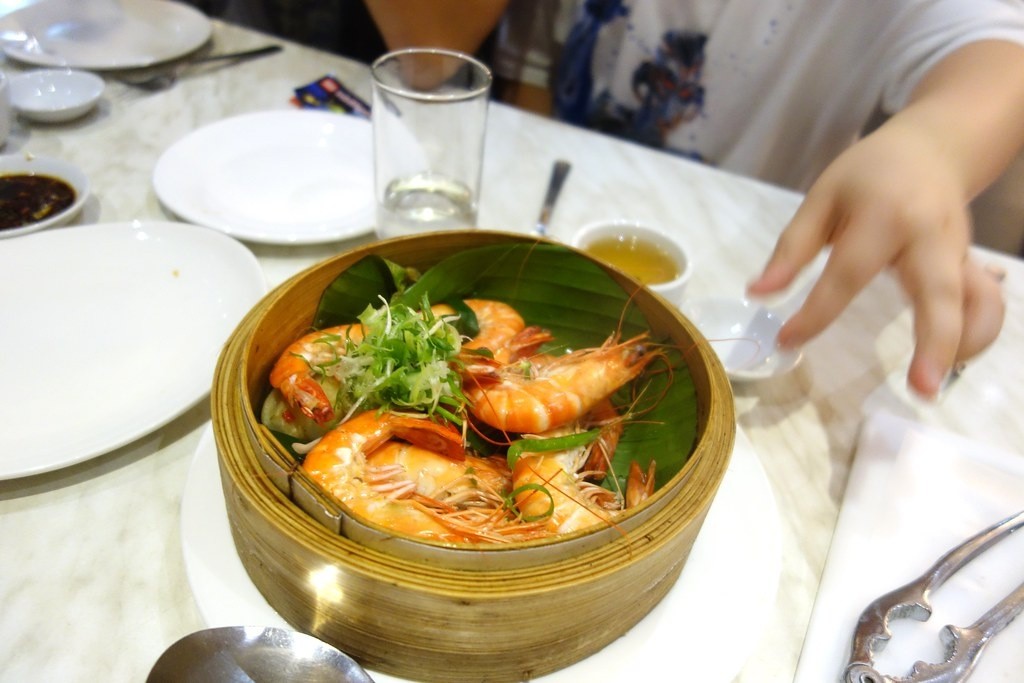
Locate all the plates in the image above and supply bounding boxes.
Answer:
[681,295,804,381]
[6,68,104,124]
[0,223,269,482]
[151,109,432,246]
[0,154,91,238]
[0,1,214,71]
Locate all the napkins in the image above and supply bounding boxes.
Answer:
[789,416,1024,683]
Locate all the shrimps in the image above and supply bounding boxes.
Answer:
[269,295,674,551]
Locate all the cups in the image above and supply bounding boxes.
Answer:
[576,222,693,311]
[370,48,493,241]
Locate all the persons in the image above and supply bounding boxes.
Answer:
[364,0,1024,399]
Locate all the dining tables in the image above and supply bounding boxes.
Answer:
[0,16,1024,683]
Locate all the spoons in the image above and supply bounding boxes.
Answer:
[116,44,282,90]
[145,626,378,683]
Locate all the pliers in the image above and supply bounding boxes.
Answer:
[839,511,1024,683]
